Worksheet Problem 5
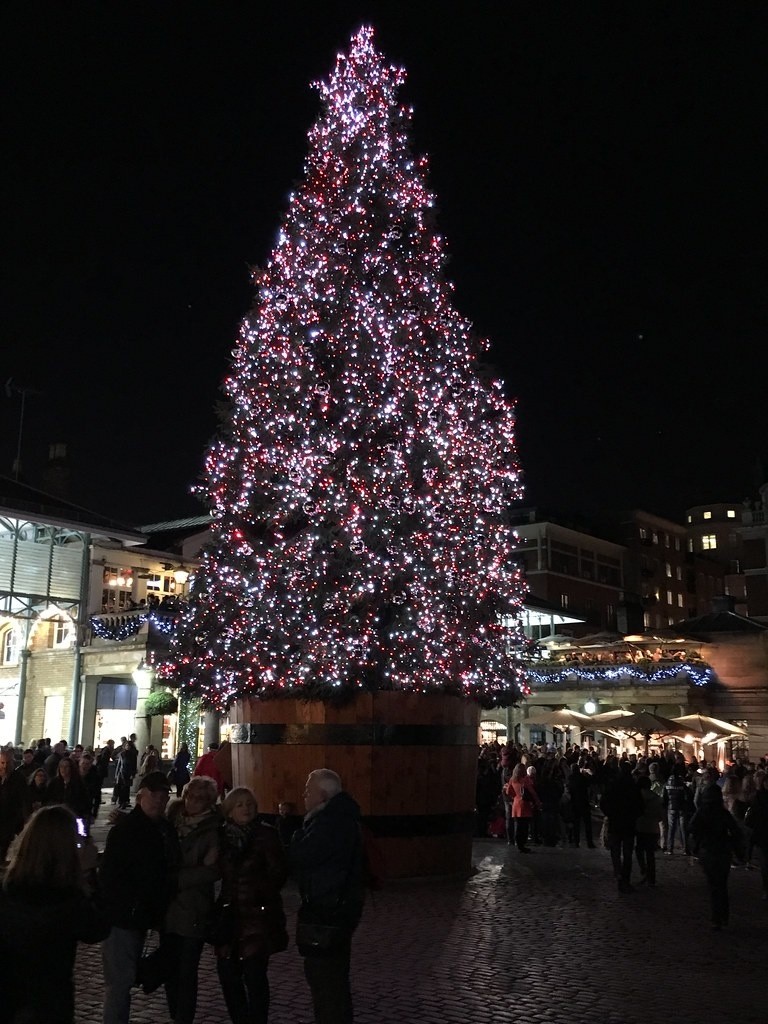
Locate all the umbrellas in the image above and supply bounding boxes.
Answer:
[521,708,748,765]
[536,628,713,654]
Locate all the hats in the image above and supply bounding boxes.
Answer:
[138,772,174,793]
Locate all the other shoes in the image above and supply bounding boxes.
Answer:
[518,844,531,854]
[664,848,675,855]
[616,876,637,892]
[745,862,752,871]
[705,907,730,924]
[586,840,598,849]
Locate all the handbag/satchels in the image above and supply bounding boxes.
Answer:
[520,786,532,801]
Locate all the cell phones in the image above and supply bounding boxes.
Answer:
[75,818,87,848]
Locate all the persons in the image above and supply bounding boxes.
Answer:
[475,740,768,871]
[539,648,705,666]
[102,593,190,635]
[0,739,114,875]
[689,785,744,931]
[280,768,376,1024]
[749,774,768,900]
[88,772,289,1024]
[600,760,665,893]
[111,734,228,810]
[0,804,115,1024]
[275,802,298,839]
